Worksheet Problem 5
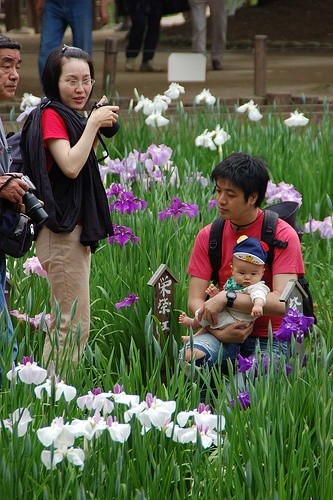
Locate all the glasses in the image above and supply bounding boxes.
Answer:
[61,79,96,86]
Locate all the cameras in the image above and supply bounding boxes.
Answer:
[17,173,49,229]
[97,103,120,140]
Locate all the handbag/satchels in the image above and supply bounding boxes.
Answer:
[0,206,32,258]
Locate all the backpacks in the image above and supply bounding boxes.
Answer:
[5,99,62,174]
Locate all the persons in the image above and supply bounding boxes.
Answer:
[178,234,269,342]
[178,154,305,416]
[117,0,191,72]
[38,0,110,99]
[0,32,45,366]
[18,44,119,388]
[186,0,227,70]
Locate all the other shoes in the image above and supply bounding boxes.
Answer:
[212,59,223,71]
[125,55,137,72]
[140,62,163,72]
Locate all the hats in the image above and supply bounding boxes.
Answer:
[232,235,270,265]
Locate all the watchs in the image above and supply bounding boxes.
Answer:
[225,289,237,308]
